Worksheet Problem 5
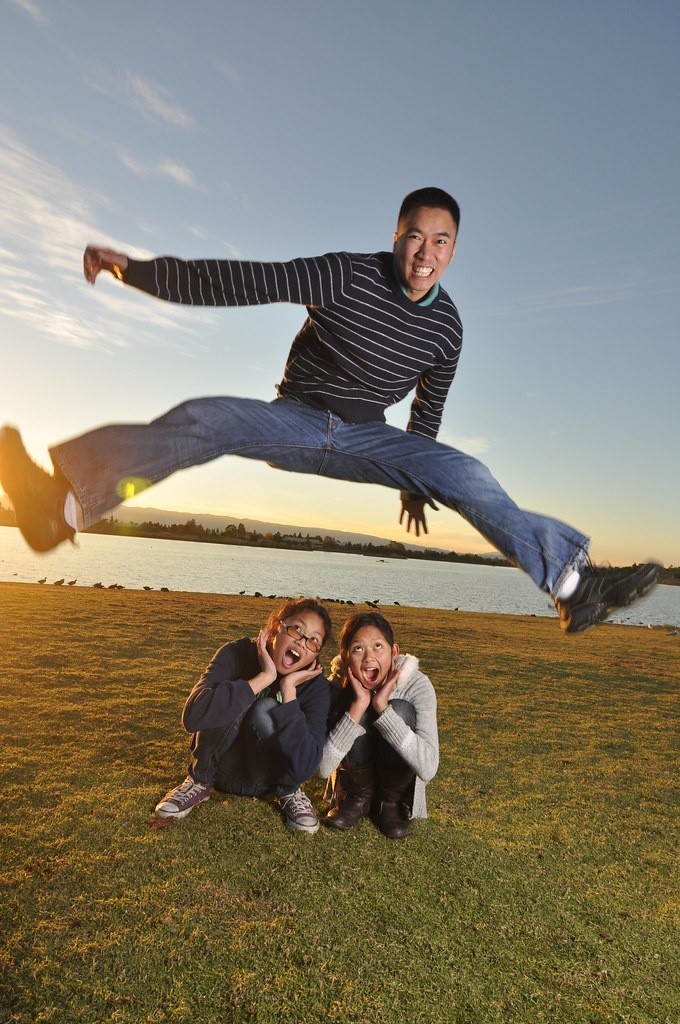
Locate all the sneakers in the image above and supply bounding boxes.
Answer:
[554,533,663,636]
[279,787,320,836]
[0,427,77,553]
[154,776,211,823]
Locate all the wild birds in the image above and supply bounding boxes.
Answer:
[454,607,459,611]
[37,575,174,592]
[240,589,402,611]
[607,616,677,636]
[531,614,536,617]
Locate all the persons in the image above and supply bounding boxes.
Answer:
[317,611,439,838]
[156,599,331,833]
[0,188,660,636]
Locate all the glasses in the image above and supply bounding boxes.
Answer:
[277,619,323,653]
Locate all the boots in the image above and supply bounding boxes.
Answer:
[326,766,374,831]
[370,778,411,838]
